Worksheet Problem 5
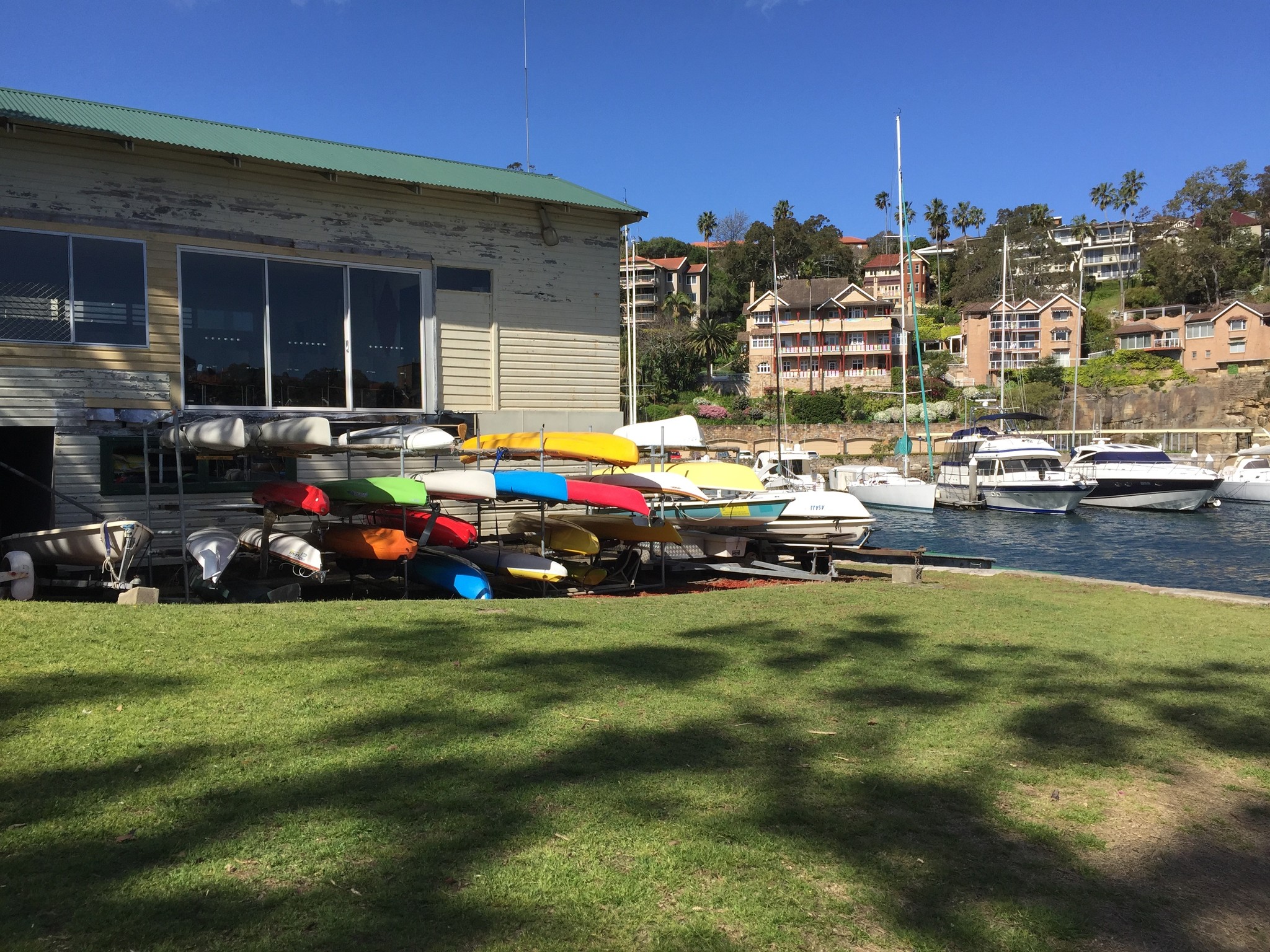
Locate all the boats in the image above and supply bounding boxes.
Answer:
[828,465,899,492]
[1218,444,1270,505]
[2,413,876,598]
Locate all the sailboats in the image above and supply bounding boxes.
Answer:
[754,235,828,492]
[1066,256,1226,512]
[843,116,939,515]
[935,223,1099,516]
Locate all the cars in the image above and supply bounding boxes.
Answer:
[739,450,754,459]
[716,452,731,459]
[808,451,820,459]
[669,450,681,461]
